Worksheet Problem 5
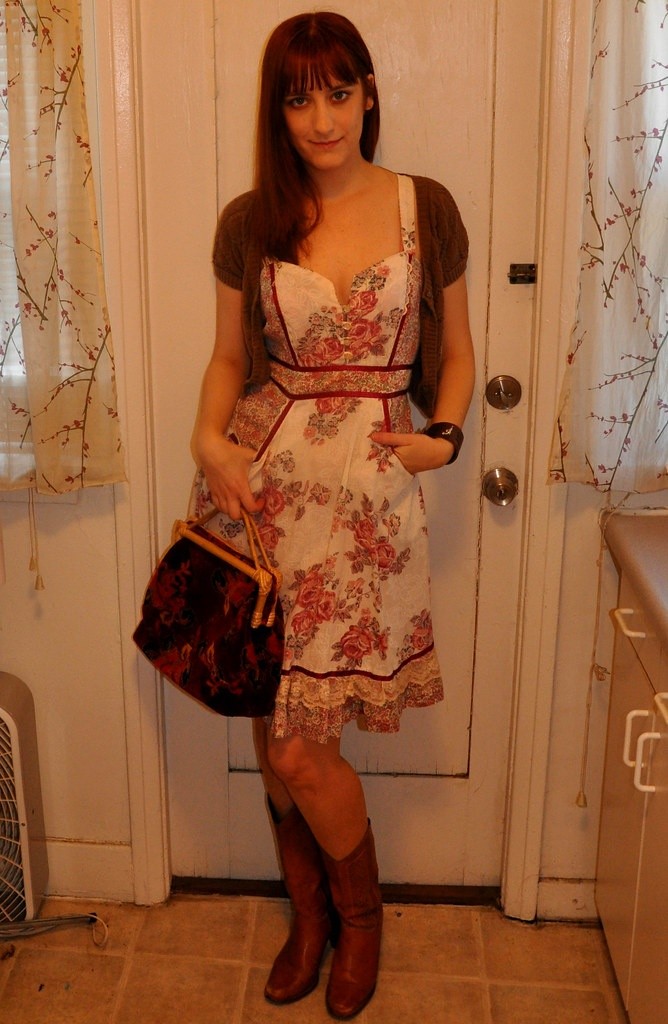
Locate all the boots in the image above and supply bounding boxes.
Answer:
[306,817,383,1020]
[263,791,342,1006]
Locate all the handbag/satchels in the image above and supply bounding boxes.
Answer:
[132,506,285,719]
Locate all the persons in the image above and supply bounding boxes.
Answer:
[187,11,475,1019]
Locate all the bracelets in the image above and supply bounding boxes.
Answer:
[423,421,464,465]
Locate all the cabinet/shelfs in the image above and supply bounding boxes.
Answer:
[595,569,668,1024]
